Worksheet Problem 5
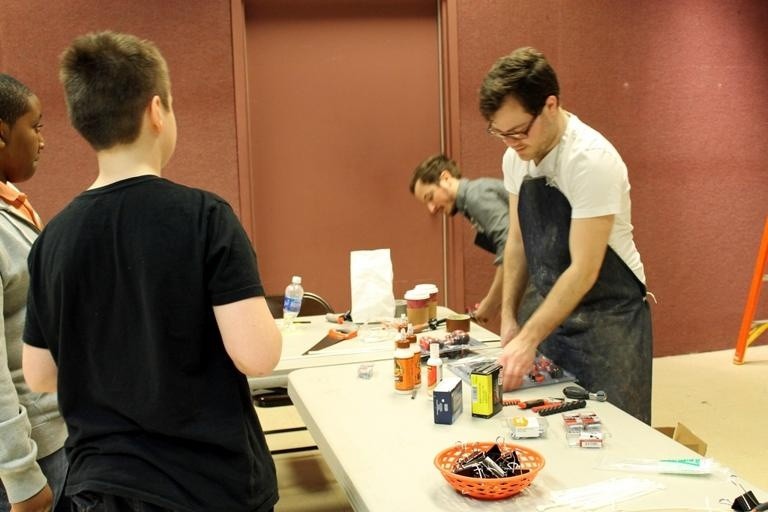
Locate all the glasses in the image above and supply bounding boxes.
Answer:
[487,112,536,140]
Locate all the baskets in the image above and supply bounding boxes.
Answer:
[434,442,545,500]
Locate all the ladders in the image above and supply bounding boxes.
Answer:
[734,216,768,365]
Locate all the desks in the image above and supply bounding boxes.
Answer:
[243,306,502,390]
[287,337,768,512]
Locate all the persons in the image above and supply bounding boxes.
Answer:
[0,72,69,512]
[20,29,283,512]
[408,153,510,326]
[475,45,653,427]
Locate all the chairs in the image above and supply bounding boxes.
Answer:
[251,293,334,455]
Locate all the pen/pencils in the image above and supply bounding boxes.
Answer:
[293,321,311,323]
[411,390,416,400]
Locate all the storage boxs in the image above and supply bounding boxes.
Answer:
[652,422,707,457]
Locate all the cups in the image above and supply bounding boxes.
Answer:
[413,284,438,322]
[403,289,429,330]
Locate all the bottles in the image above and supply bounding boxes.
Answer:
[426,343,443,401]
[282,275,304,319]
[394,324,422,394]
